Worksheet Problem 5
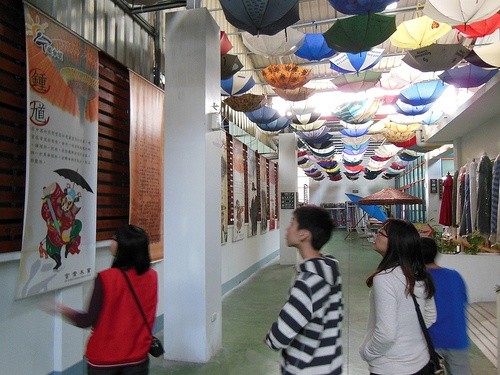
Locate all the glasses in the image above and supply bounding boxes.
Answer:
[111,234,118,241]
[377,227,388,239]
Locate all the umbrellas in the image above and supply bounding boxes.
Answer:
[218,0,500,181]
[52,168,96,194]
[358,188,424,218]
[344,193,389,240]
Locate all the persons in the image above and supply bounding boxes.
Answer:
[358,217,437,375]
[44,225,160,375]
[264,206,344,375]
[420,237,472,375]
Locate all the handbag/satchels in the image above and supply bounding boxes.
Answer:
[149,335,164,358]
[431,350,452,375]
